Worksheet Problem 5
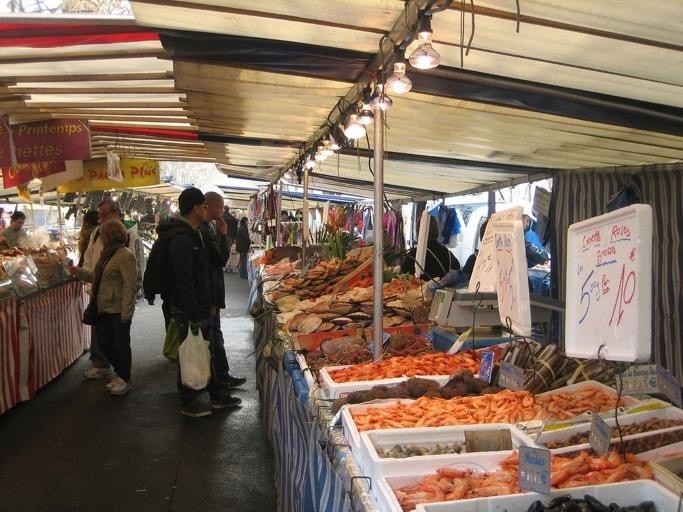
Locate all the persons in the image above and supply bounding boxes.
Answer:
[222,204,303,272]
[80,198,143,381]
[139,208,155,223]
[90,219,135,395]
[401,213,461,282]
[0,207,6,232]
[78,210,101,269]
[1,210,40,256]
[521,214,549,268]
[143,187,251,419]
[418,218,534,300]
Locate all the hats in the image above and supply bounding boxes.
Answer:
[178,188,204,212]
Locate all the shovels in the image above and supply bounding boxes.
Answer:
[518,410,593,428]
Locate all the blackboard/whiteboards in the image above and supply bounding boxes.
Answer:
[467,206,535,339]
[564,202,653,362]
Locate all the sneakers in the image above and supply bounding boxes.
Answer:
[211,397,242,408]
[110,379,133,395]
[83,368,113,378]
[180,404,212,417]
[106,377,118,389]
[228,373,247,389]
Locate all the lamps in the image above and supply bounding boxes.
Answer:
[304,8,442,172]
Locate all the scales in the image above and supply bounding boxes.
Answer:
[427,288,504,331]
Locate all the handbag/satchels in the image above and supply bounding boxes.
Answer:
[84,304,97,325]
